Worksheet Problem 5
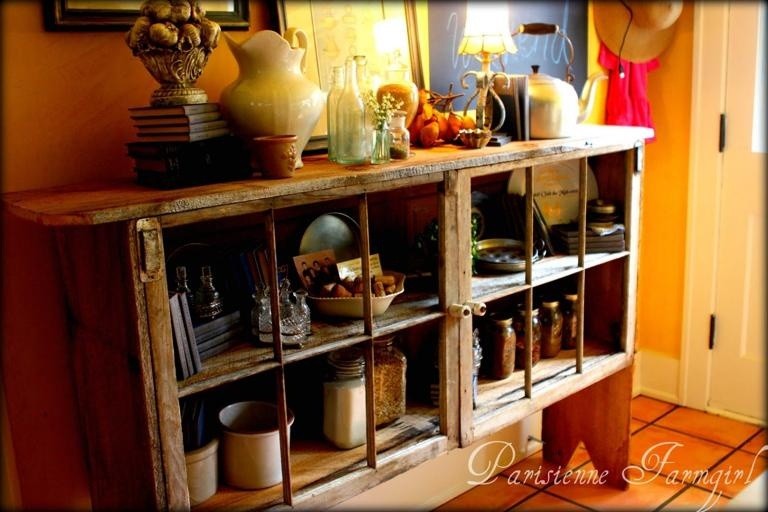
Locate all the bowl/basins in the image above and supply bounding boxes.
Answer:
[310,271,405,319]
[296,211,373,264]
[458,125,493,149]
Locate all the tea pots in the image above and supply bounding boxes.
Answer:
[219,25,328,172]
[496,21,608,140]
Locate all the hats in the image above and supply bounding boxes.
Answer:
[592,0,684,64]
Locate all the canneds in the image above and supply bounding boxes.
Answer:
[322,332,407,450]
[488,292,578,380]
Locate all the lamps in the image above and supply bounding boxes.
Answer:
[457,0,518,133]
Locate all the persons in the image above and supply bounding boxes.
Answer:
[302,257,335,292]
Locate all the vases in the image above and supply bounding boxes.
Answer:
[369,128,393,165]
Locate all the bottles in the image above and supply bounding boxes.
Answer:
[338,60,367,166]
[386,112,412,162]
[248,279,312,348]
[173,266,197,324]
[347,54,373,162]
[199,263,222,319]
[327,64,351,162]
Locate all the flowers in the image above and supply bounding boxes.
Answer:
[358,88,405,127]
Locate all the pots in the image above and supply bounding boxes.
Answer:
[470,233,548,274]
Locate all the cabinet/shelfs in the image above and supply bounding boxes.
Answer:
[1,123,655,512]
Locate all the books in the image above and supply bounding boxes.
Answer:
[127,102,229,158]
[552,224,626,254]
[168,290,241,381]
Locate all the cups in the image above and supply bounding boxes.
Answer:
[251,133,299,178]
[366,127,390,165]
[176,425,218,506]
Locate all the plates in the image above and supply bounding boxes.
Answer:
[506,158,599,227]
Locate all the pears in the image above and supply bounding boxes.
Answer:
[407,88,476,147]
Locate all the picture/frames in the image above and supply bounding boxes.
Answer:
[41,0,249,33]
[277,0,425,151]
